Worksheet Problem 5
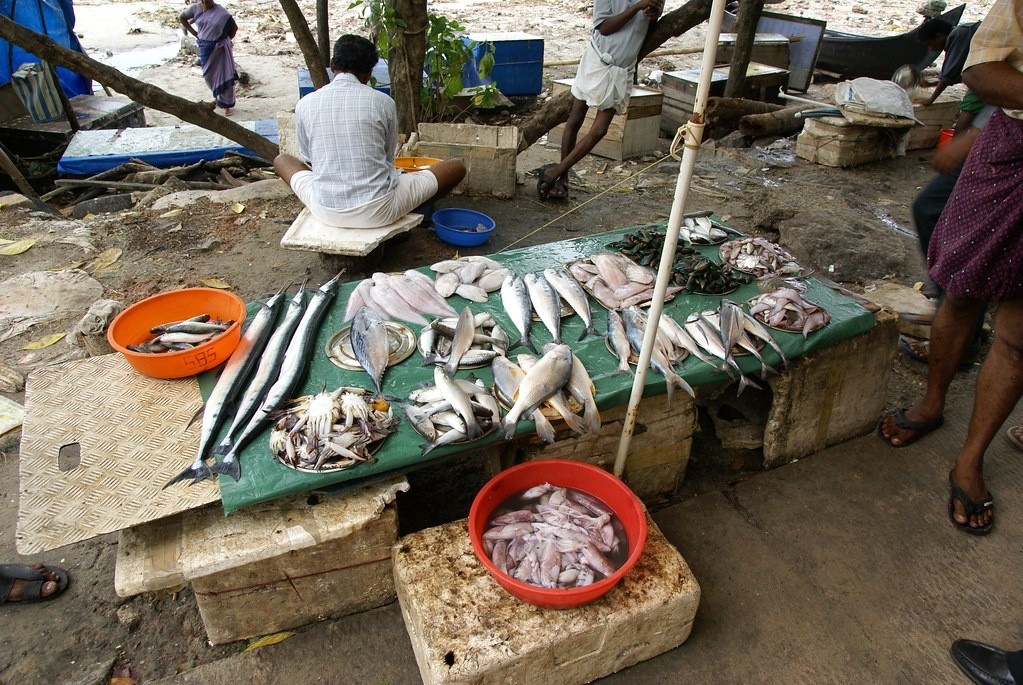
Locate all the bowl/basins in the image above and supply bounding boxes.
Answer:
[432,208,496,246]
[107,287,247,379]
[469,460,647,610]
[394,156,443,173]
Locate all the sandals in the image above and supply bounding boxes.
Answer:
[0,562,68,605]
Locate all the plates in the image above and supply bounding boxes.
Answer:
[269,232,832,473]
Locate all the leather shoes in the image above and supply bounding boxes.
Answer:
[950,638,1017,685]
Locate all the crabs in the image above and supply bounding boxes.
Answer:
[261,380,401,473]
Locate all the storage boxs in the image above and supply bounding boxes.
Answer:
[907,90,962,150]
[548,78,664,161]
[797,116,916,168]
[0,94,146,187]
[660,60,791,137]
[714,33,790,70]
[278,107,311,161]
[298,32,544,99]
[404,123,518,199]
[114,284,901,685]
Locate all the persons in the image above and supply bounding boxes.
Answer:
[179,0,238,116]
[537,1,665,196]
[878,0,1023,535]
[274,34,467,230]
[950,637,1023,685]
[0,564,70,606]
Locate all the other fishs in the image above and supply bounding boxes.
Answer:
[209,275,311,456]
[125,312,233,356]
[188,268,345,486]
[341,209,831,456]
[161,280,293,491]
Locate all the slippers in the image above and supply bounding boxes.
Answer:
[1006,426,1023,451]
[223,108,233,116]
[900,336,977,371]
[948,467,994,535]
[878,407,945,448]
[537,168,569,201]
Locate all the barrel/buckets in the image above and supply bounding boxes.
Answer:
[937,129,955,146]
